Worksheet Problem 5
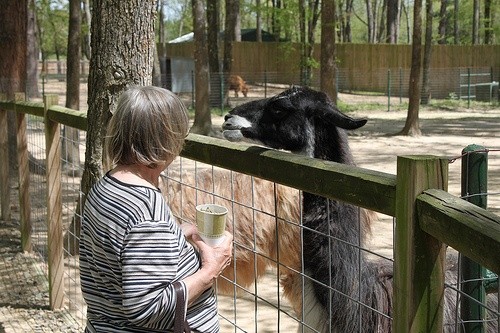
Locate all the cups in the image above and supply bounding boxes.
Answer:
[196,204,228,247]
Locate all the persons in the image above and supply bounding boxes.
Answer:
[79,84,234,333]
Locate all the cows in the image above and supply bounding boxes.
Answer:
[227,75,249,97]
